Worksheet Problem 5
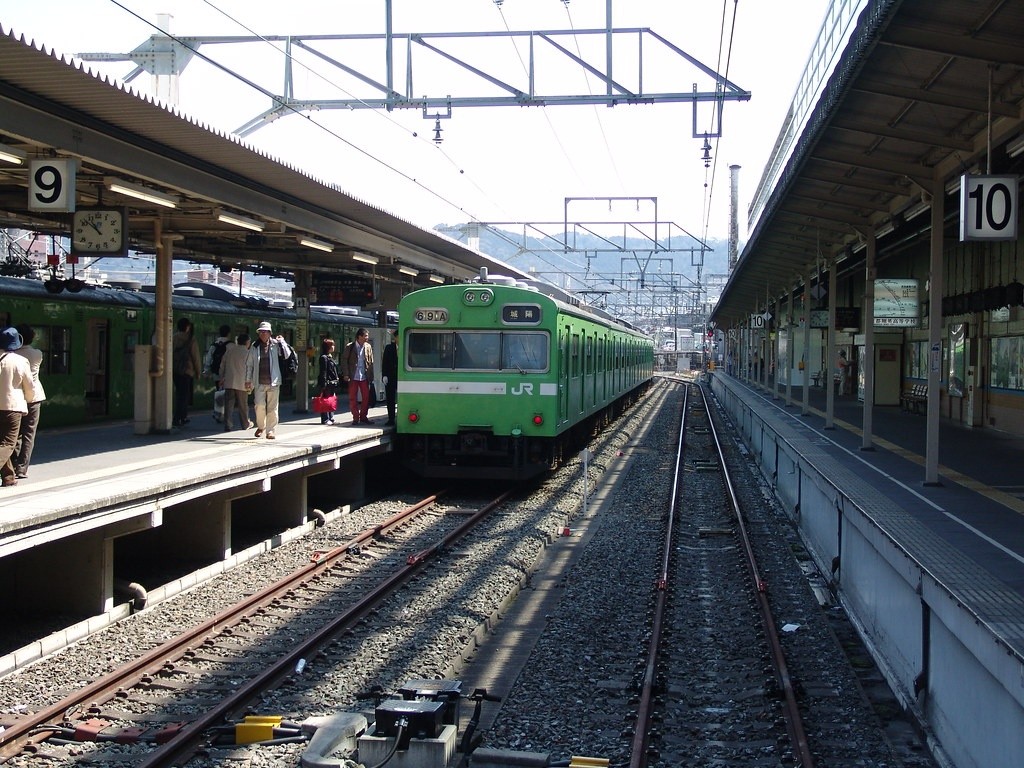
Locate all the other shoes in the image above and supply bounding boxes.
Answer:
[242,421,254,430]
[255,426,266,437]
[333,421,341,425]
[2,480,18,486]
[15,473,28,478]
[266,434,275,439]
[321,420,333,425]
[173,420,181,426]
[360,417,375,424]
[352,420,367,426]
[385,420,395,425]
[181,419,190,426]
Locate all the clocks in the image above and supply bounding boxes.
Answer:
[809,300,829,328]
[70,185,130,258]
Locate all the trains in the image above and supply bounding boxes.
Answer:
[398,267,654,490]
[1,273,397,432]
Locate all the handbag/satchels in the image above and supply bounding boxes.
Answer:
[278,341,298,376]
[213,384,226,422]
[312,390,336,412]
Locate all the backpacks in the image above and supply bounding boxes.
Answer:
[210,341,235,374]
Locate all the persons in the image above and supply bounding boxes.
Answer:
[10,326,47,478]
[0,326,35,487]
[382,330,399,425]
[726,351,732,376]
[317,339,340,425]
[246,322,290,441]
[340,329,375,425]
[749,351,762,379]
[838,349,854,396]
[219,335,254,432]
[173,317,201,427]
[202,324,235,424]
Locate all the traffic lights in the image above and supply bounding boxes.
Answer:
[708,330,713,337]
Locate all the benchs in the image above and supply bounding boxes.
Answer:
[810,370,842,391]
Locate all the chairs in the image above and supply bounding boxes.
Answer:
[899,384,928,416]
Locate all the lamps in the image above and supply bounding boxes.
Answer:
[214,209,266,232]
[349,251,379,265]
[429,275,445,284]
[296,234,334,253]
[103,176,179,208]
[397,265,420,277]
[0,143,27,165]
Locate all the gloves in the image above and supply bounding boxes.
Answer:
[383,376,388,385]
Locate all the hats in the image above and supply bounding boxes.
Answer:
[256,322,272,333]
[0,327,23,351]
